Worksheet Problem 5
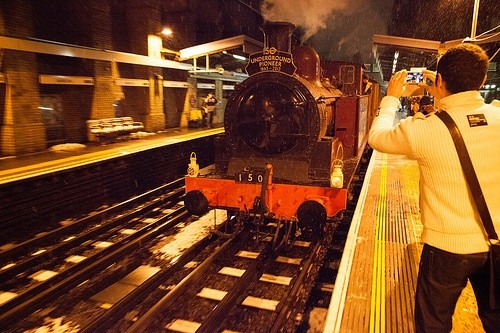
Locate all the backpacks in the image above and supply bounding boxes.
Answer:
[488,244,500,312]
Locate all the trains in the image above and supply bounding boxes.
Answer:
[185,16,382,235]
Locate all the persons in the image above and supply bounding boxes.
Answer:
[406,71,423,83]
[366,43,500,331]
[381,88,434,117]
[189,92,219,128]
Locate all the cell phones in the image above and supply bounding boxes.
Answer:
[405,71,427,85]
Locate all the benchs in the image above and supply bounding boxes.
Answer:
[86,115,145,145]
